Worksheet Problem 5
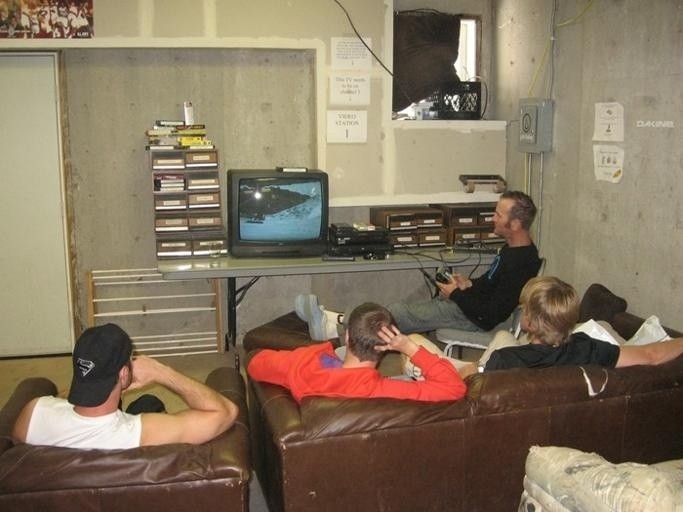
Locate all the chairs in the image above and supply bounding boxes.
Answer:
[437,258,546,360]
[1,366,254,511]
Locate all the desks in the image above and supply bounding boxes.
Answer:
[156,249,496,354]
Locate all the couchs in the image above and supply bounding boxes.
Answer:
[242,312,682,512]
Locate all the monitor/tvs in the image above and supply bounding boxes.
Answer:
[226,168,329,258]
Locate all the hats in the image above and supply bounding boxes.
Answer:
[67,324,133,406]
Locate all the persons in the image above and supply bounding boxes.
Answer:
[294,190,542,339]
[13,323,239,454]
[246,302,469,407]
[479,275,683,372]
[0,0,94,39]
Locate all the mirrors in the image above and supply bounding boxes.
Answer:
[394,12,482,120]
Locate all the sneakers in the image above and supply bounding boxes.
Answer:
[295,292,328,341]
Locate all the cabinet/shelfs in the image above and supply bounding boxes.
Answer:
[147,147,228,260]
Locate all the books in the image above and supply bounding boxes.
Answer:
[144,119,215,151]
[160,179,184,192]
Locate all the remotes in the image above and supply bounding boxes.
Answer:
[322,256,355,261]
